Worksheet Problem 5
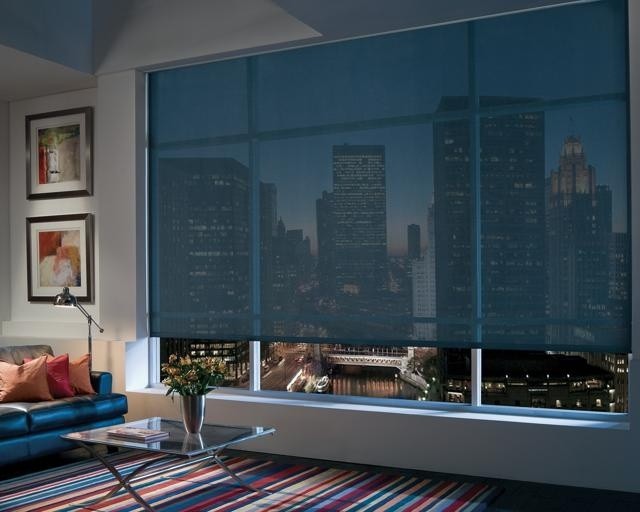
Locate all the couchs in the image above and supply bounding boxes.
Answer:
[0,344,128,469]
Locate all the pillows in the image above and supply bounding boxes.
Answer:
[0,351,98,403]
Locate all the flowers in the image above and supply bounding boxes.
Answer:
[159,352,230,404]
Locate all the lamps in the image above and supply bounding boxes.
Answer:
[53,288,105,370]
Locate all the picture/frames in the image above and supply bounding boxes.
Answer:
[24,106,95,304]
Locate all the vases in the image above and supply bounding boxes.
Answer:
[181,395,206,433]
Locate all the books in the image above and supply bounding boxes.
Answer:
[107,435,170,444]
[104,424,172,441]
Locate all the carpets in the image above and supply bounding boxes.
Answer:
[0,449,505,512]
